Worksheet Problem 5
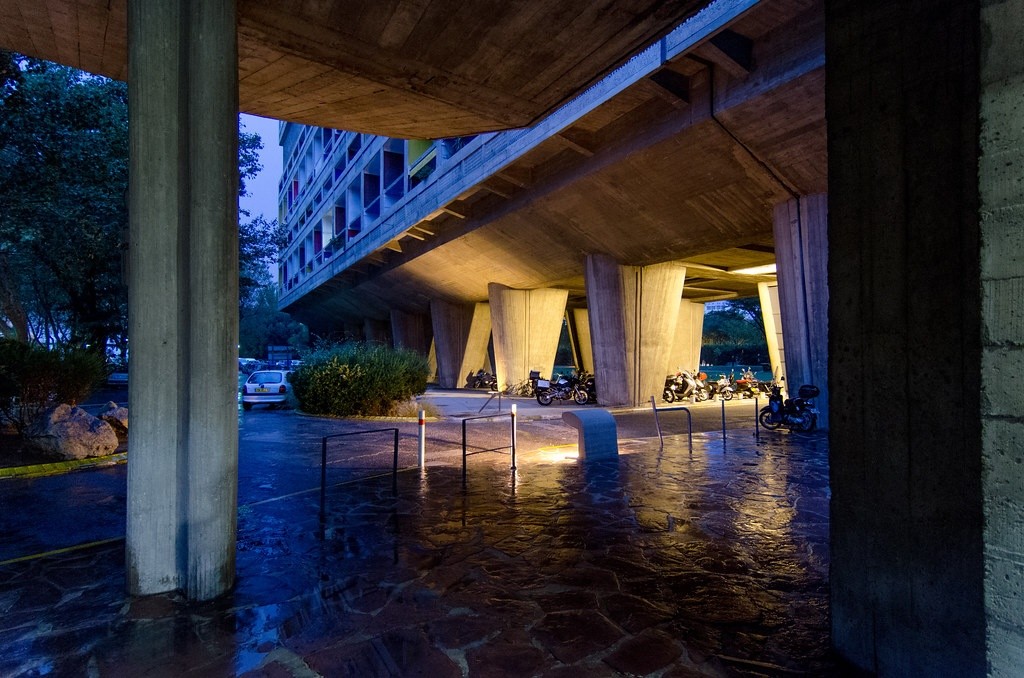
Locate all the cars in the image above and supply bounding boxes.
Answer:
[237,357,270,375]
[101,372,129,391]
[242,370,298,410]
[279,358,307,367]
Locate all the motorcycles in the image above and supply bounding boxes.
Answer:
[662,368,716,403]
[716,366,760,401]
[759,376,820,433]
[529,370,596,405]
[473,367,498,391]
